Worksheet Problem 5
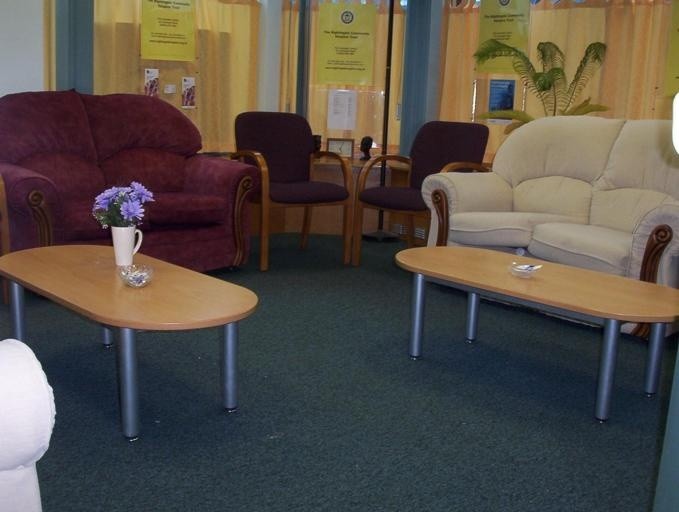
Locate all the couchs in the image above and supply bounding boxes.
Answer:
[0,87,262,293]
[419,115,679,343]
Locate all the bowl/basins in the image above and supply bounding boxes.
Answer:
[117,264,155,290]
[508,258,544,279]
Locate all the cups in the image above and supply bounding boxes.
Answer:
[312,134,322,162]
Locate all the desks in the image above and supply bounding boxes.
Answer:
[0,244,260,444]
[395,246,678,423]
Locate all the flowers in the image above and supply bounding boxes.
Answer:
[92,181,156,230]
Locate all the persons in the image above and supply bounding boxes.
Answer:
[146,77,159,98]
[184,85,195,107]
[497,83,514,110]
[360,135,373,156]
[312,135,322,150]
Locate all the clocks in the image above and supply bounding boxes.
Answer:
[326,138,355,162]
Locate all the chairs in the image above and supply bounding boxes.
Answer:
[352,121,490,267]
[234,111,355,272]
